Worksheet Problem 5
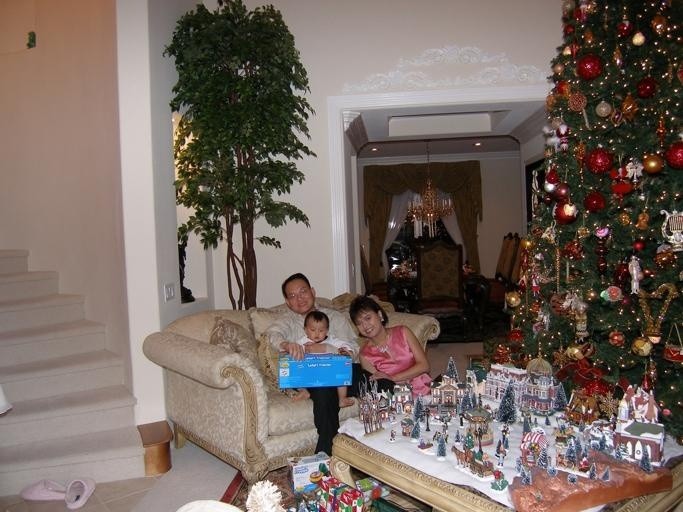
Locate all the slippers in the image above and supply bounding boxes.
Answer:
[19,476,67,502]
[62,475,96,511]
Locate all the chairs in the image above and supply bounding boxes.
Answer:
[466,232,525,323]
[412,240,465,335]
[142,296,442,495]
[360,245,399,312]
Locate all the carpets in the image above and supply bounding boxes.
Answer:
[220,467,300,512]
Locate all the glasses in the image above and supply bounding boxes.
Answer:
[285,287,309,300]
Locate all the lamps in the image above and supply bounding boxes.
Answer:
[407,140,456,239]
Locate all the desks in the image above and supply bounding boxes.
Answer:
[388,269,491,333]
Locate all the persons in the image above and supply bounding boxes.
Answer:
[349,295,436,399]
[294,310,355,407]
[266,273,396,457]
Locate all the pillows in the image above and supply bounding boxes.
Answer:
[210,316,267,385]
[248,306,282,343]
